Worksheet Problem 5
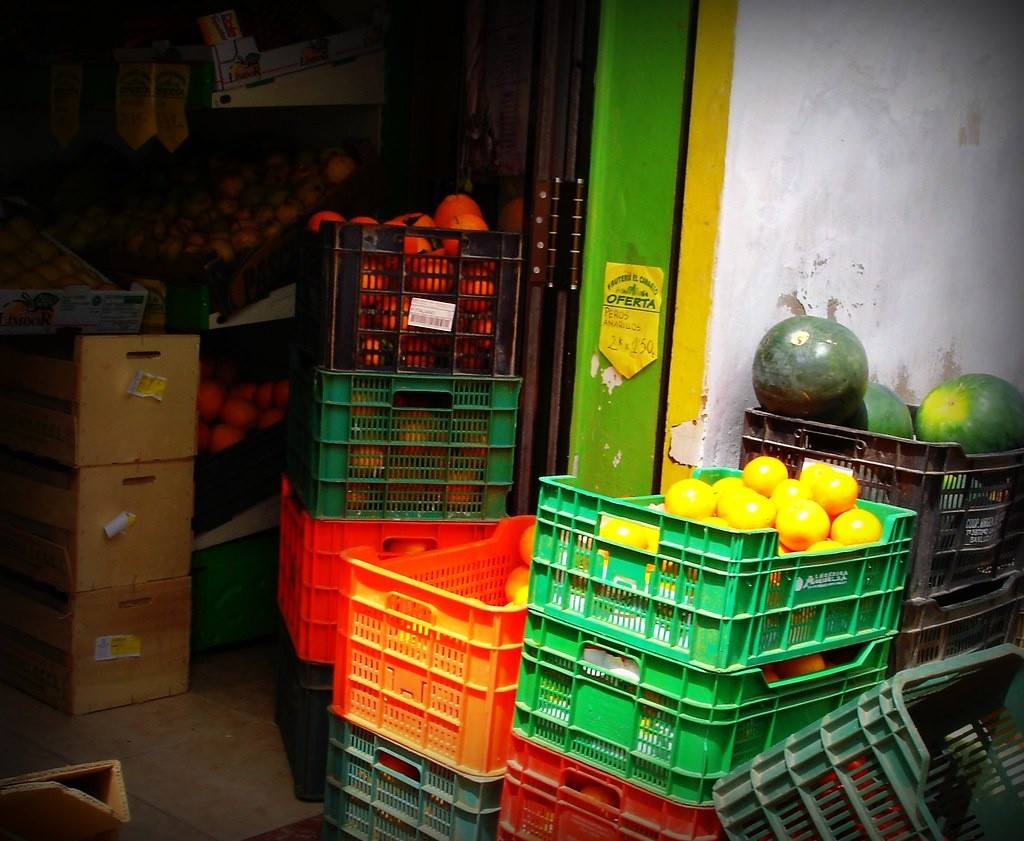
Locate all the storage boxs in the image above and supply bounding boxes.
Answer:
[2,0,1024,838]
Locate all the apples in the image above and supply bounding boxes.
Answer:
[0,147,356,291]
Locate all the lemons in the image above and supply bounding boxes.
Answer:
[502,457,883,681]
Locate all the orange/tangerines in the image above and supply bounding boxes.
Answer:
[198,194,498,515]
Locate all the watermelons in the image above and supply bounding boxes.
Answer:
[751,315,1024,455]
